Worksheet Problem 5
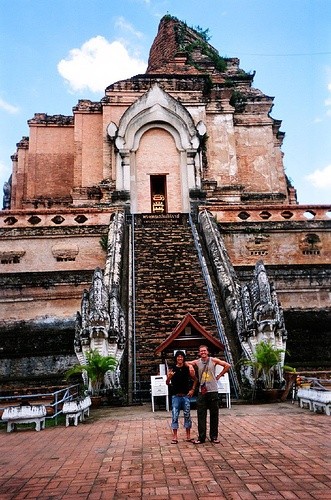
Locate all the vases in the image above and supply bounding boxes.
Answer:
[300,382,312,390]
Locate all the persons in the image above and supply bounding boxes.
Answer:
[166,351,198,444]
[185,345,231,444]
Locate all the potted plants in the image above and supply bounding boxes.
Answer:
[62,348,119,409]
[232,337,292,404]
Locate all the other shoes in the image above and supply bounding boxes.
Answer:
[171,439,178,444]
[211,438,220,443]
[185,438,194,442]
[194,439,205,444]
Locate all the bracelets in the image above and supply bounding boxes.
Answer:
[192,386,195,389]
